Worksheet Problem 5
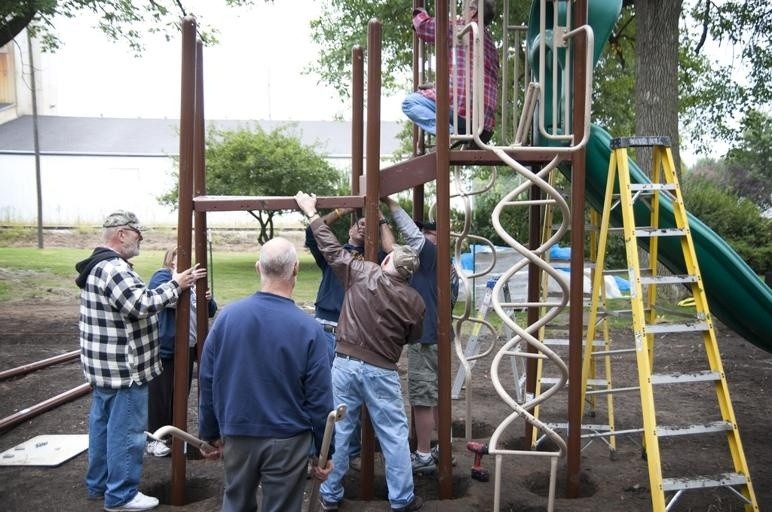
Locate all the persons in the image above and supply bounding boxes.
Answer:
[73,207,202,512]
[292,190,427,511]
[304,207,366,481]
[145,243,218,458]
[196,238,338,511]
[402,1,500,150]
[377,195,460,477]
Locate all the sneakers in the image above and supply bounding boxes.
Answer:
[391,495,424,512]
[348,455,361,472]
[317,493,338,511]
[146,440,171,457]
[104,491,159,512]
[409,451,437,476]
[431,446,457,466]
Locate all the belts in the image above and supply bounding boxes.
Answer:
[336,353,361,361]
[322,324,334,333]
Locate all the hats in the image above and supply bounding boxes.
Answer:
[413,220,436,230]
[391,242,420,278]
[103,210,150,231]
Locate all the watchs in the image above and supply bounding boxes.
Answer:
[305,211,319,219]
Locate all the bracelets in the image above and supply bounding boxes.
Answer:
[379,218,392,225]
[335,208,341,219]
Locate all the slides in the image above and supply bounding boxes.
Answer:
[555,124,772,354]
